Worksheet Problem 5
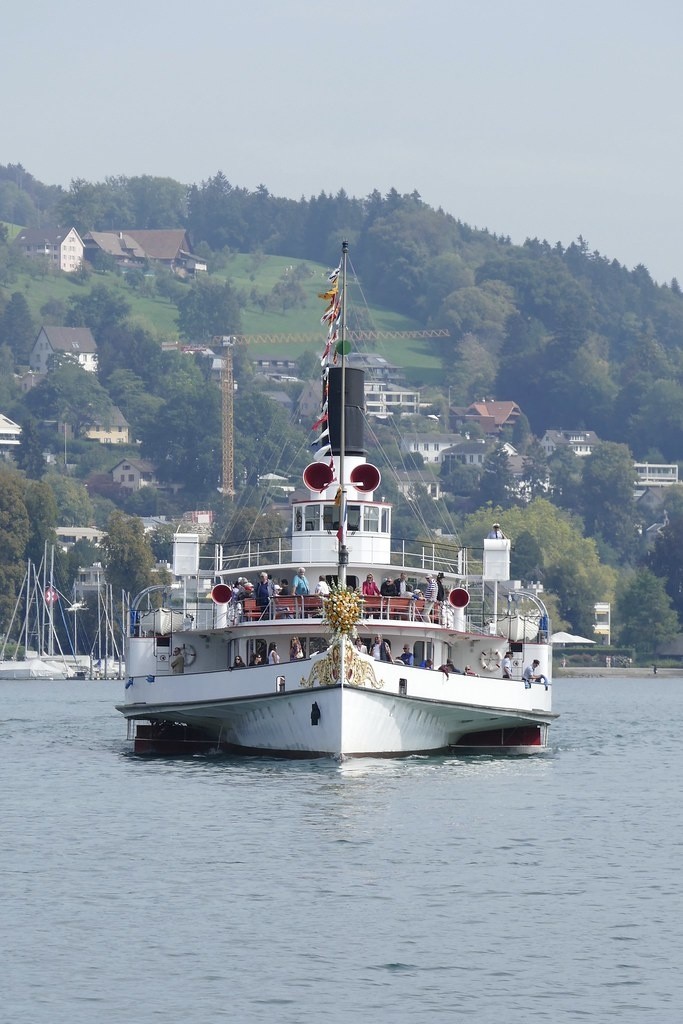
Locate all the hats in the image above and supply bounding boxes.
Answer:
[437,573,445,578]
[534,660,540,664]
[492,523,500,527]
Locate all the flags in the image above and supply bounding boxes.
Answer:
[311,269,353,507]
[45,587,58,605]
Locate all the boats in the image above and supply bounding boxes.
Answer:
[114,239,560,758]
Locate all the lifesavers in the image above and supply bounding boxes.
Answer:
[172,643,197,667]
[478,647,502,673]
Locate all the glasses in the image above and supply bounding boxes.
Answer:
[235,659,240,660]
[258,657,263,659]
[426,578,429,579]
[367,577,372,579]
[386,581,391,583]
[292,639,296,641]
[403,648,407,649]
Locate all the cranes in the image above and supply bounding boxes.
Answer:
[210,328,450,509]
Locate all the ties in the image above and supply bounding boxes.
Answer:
[495,532,497,538]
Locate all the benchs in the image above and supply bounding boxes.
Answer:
[244,595,439,622]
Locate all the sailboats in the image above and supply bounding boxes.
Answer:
[0,540,129,681]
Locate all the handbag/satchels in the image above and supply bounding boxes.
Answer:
[297,652,304,658]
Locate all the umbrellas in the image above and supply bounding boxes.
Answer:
[550,632,596,668]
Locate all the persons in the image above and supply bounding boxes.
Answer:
[487,523,503,539]
[465,665,480,677]
[419,659,433,669]
[422,573,446,623]
[363,573,421,618]
[268,642,280,664]
[353,637,367,654]
[171,647,184,673]
[522,660,541,681]
[248,654,263,666]
[370,635,394,664]
[446,658,461,673]
[501,652,513,679]
[228,656,246,667]
[290,636,301,661]
[438,664,454,679]
[231,568,329,621]
[400,643,413,667]
